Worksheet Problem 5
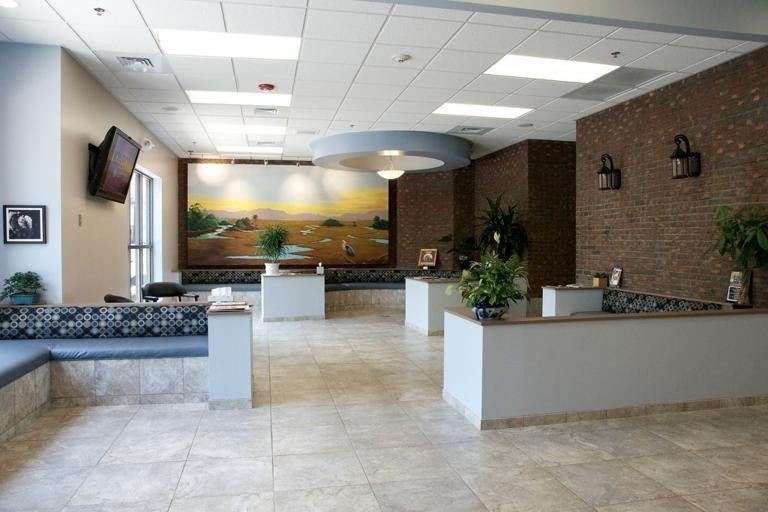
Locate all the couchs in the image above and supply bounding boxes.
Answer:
[1,305,250,444]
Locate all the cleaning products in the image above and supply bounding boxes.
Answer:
[316,261,325,274]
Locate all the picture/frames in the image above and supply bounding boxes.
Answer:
[4,203,47,243]
[174,158,398,270]
[417,248,437,267]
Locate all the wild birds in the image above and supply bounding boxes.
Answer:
[341,239,356,258]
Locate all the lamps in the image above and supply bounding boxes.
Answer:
[597,153,622,191]
[670,134,702,178]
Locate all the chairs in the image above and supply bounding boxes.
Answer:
[142,282,199,306]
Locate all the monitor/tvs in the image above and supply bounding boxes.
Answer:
[87,125,142,204]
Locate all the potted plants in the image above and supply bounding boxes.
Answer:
[712,199,768,308]
[592,272,608,288]
[1,270,47,305]
[447,256,532,324]
[254,222,289,276]
